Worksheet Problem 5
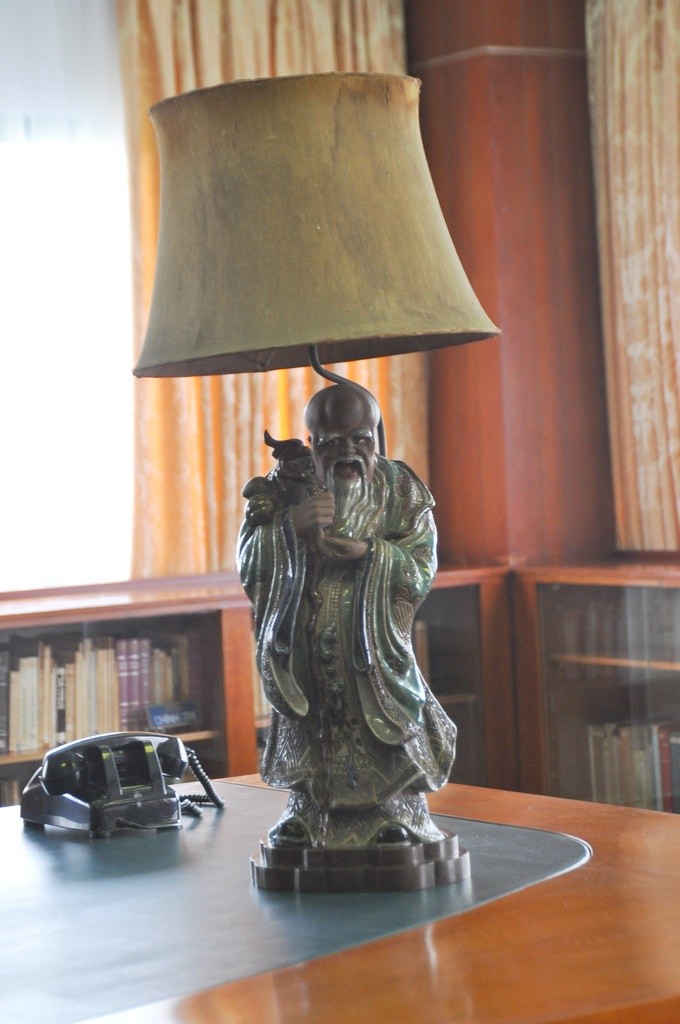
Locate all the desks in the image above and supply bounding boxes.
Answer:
[0,773,679,1023]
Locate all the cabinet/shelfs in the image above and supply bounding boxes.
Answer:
[2,554,512,787]
[509,548,677,813]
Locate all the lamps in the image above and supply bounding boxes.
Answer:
[127,73,503,892]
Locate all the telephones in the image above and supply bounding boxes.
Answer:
[18,730,190,841]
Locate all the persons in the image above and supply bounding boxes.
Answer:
[235,380,477,896]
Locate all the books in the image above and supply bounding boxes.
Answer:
[570,581,680,816]
[0,622,224,807]
